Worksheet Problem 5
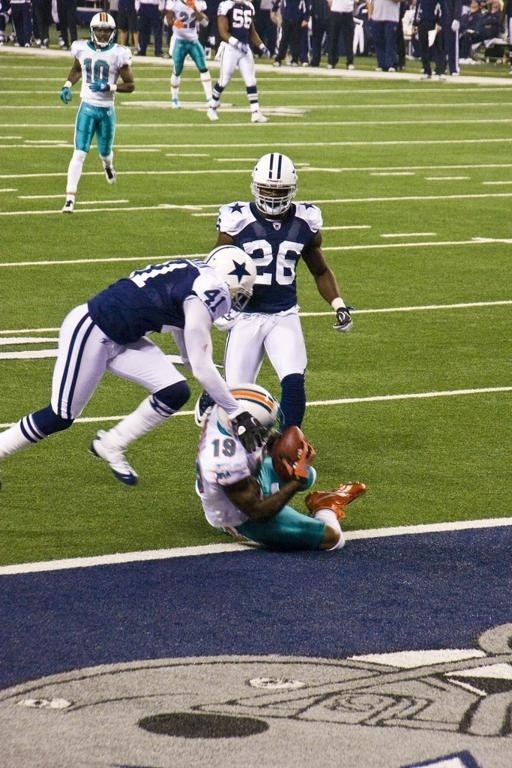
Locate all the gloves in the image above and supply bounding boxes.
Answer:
[232,410,268,453]
[282,439,316,483]
[89,81,112,93]
[58,86,74,104]
[173,1,195,28]
[332,298,355,332]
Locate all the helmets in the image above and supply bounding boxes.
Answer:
[90,12,118,49]
[203,244,257,296]
[217,384,280,440]
[248,152,299,216]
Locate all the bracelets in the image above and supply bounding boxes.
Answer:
[331,297,346,311]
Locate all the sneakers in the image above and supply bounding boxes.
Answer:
[61,194,78,214]
[304,480,367,518]
[171,95,269,122]
[193,387,215,427]
[14,37,78,50]
[88,430,140,486]
[104,163,116,184]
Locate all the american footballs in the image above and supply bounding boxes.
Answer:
[272,425,311,483]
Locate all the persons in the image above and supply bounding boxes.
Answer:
[194,152,354,435]
[194,384,367,552]
[1,245,268,484]
[60,12,135,212]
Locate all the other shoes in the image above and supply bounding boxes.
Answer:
[135,45,169,59]
[260,46,457,84]
